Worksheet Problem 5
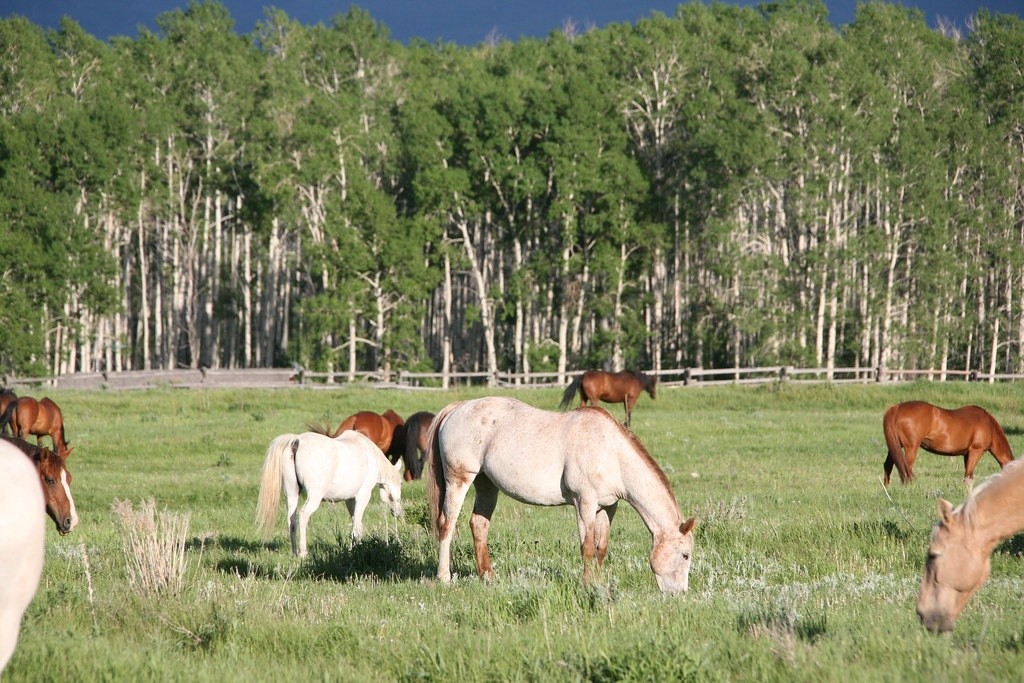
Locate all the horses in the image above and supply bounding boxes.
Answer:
[884,399,1024,634]
[249,366,699,600]
[0,385,78,683]
